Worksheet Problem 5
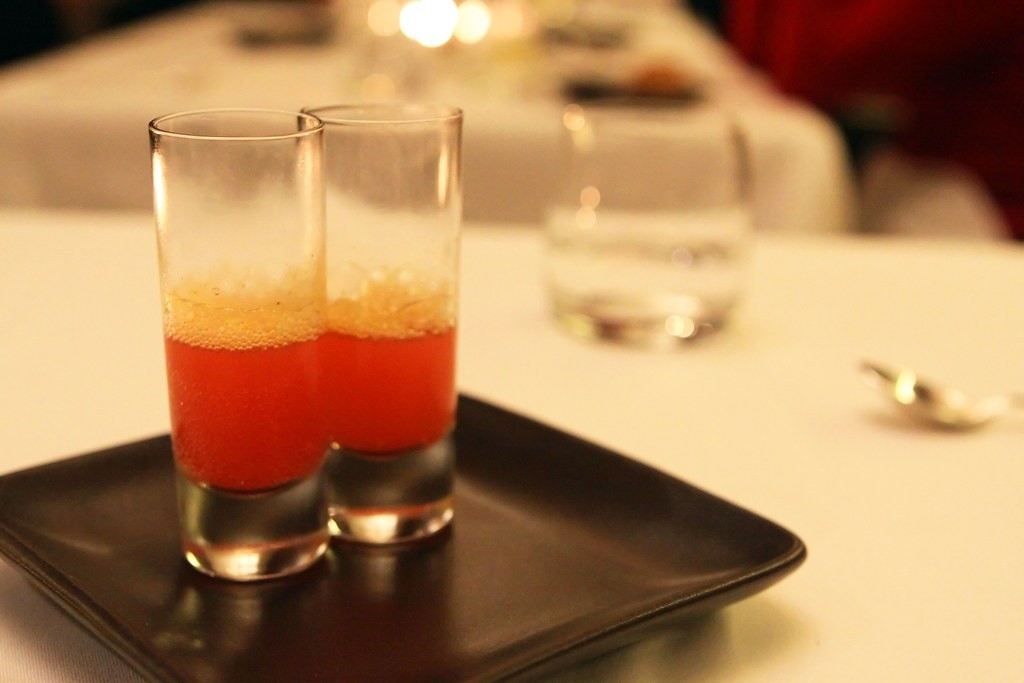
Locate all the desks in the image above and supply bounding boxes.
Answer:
[0,212,1024,683]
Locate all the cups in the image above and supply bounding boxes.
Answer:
[539,104,760,356]
[148,109,331,583]
[299,103,464,546]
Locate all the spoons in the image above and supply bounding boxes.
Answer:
[860,349,1004,433]
